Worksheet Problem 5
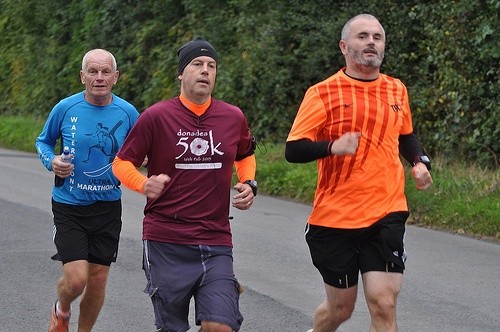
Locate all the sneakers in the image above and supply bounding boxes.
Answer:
[48,299,71,332]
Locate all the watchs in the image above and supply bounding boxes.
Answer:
[411,155,432,171]
[242,180,258,196]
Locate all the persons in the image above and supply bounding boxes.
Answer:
[284,14,433,332]
[34,49,148,332]
[112,37,258,332]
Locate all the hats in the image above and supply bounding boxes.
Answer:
[177,37,218,75]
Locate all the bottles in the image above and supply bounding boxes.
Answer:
[59,146,72,163]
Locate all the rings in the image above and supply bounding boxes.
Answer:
[247,201,251,206]
[60,171,62,174]
